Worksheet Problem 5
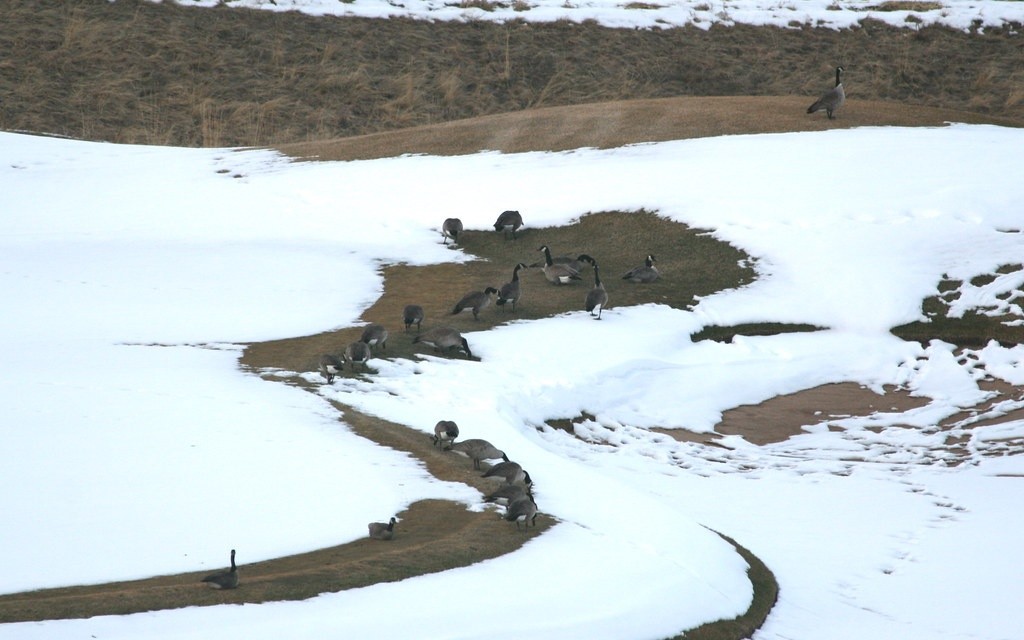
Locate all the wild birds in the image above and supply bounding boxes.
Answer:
[482,463,533,488]
[360,324,388,350]
[429,421,459,447]
[493,210,523,241]
[450,287,498,320]
[414,326,472,359]
[807,64,849,119]
[505,494,538,529]
[481,485,534,508]
[319,354,343,383]
[444,439,510,470]
[498,263,526,314]
[530,246,583,285]
[442,218,463,244]
[345,342,369,370]
[368,517,397,540]
[576,253,608,320]
[401,304,424,332]
[621,254,659,284]
[201,549,240,589]
